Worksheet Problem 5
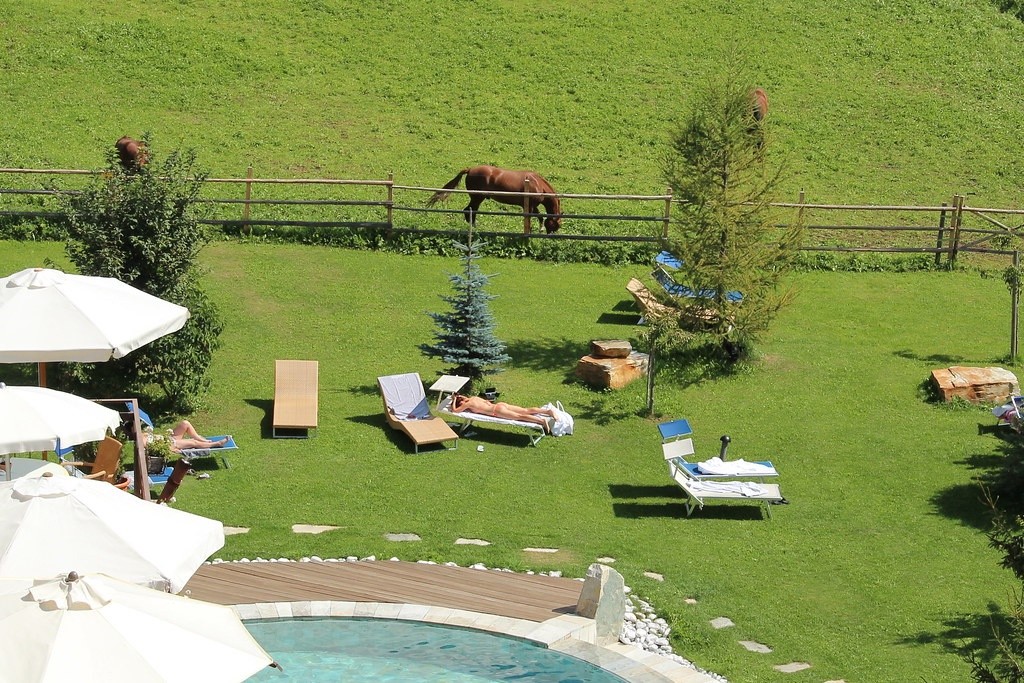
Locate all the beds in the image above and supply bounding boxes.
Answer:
[429,375,553,446]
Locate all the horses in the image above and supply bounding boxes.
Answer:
[425,163,564,235]
[114,134,152,176]
[738,88,770,161]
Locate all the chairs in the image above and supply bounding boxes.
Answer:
[627,278,736,332]
[662,438,782,519]
[650,251,745,307]
[120,423,237,471]
[273,359,318,439]
[377,373,459,454]
[658,418,779,483]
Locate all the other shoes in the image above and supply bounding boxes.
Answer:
[187,469,197,476]
[198,473,210,480]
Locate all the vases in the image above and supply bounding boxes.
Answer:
[145,451,165,473]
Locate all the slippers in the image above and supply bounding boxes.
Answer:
[477,444,484,452]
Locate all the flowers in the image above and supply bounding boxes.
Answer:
[144,425,178,462]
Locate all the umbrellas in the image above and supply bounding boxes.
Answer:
[1,267,282,682]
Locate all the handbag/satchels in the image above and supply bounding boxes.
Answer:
[540,400,574,436]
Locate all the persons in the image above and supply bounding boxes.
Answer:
[451,393,559,433]
[129,420,230,449]
